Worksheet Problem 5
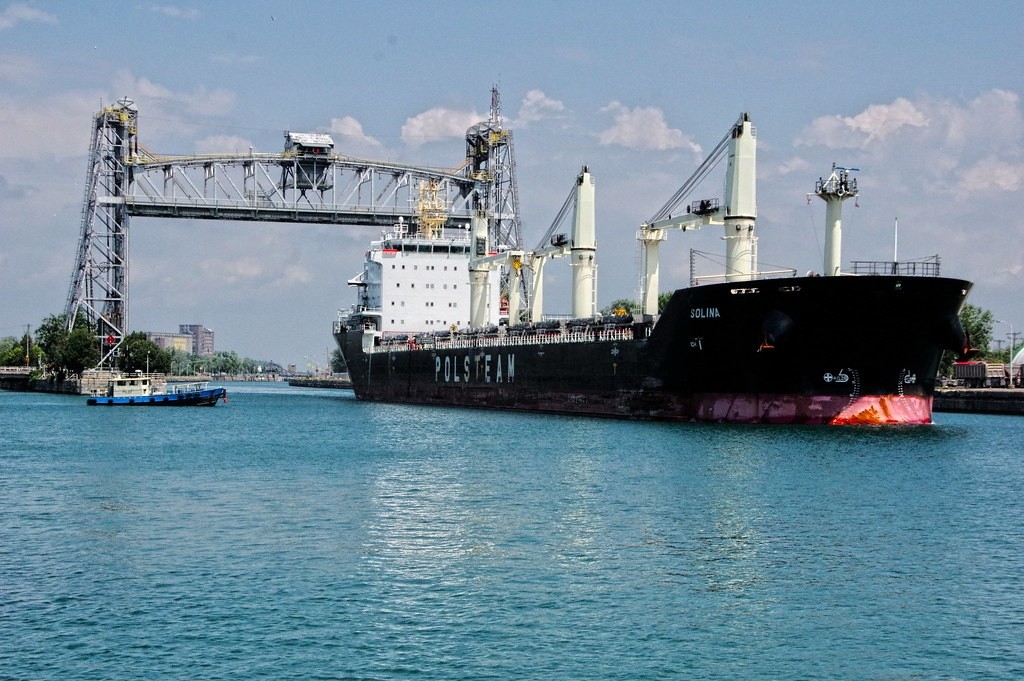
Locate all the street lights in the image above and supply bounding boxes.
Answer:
[991,315,1015,389]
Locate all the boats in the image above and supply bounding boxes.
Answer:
[83,375,229,410]
[327,81,975,432]
